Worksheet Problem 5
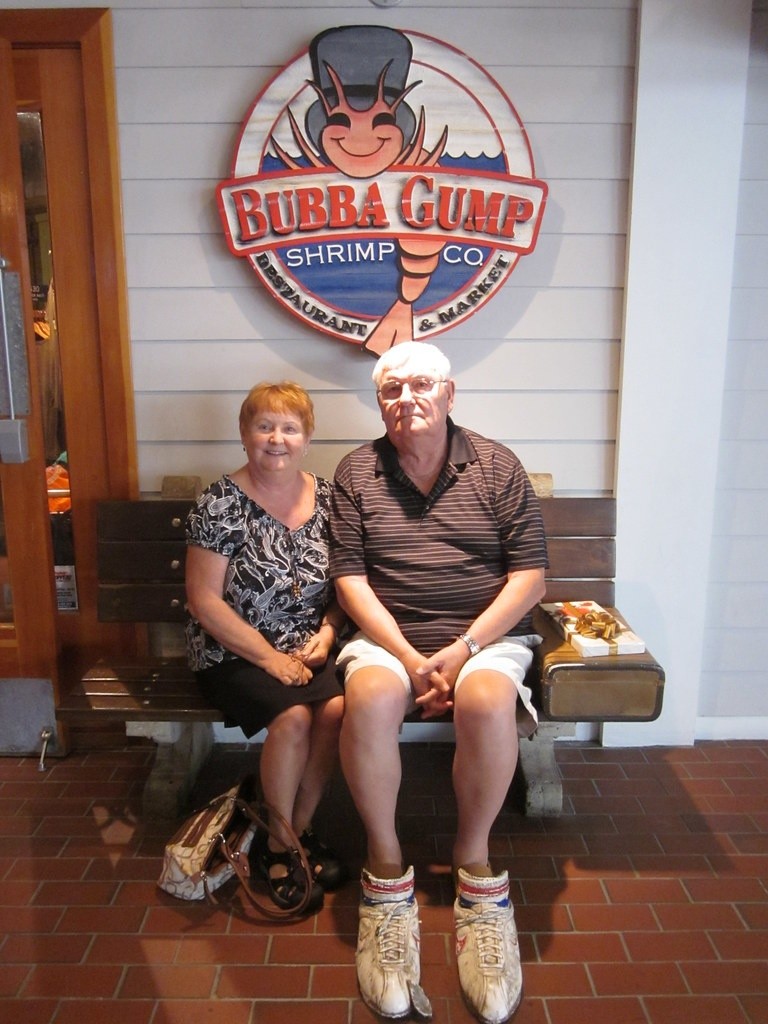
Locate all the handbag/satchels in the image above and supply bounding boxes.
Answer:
[155,778,311,917]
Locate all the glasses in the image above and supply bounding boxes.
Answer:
[377,379,448,400]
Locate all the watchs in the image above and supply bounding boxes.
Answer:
[459,632,481,659]
[320,622,340,644]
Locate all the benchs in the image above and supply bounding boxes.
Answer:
[55,475,665,818]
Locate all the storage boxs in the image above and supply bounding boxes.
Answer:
[533,606,665,722]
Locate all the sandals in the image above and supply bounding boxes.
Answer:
[258,841,325,911]
[294,827,349,890]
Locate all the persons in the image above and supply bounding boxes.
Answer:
[328,340,548,1024]
[183,381,347,917]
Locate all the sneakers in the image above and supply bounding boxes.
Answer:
[355,862,421,1019]
[452,867,523,1023]
